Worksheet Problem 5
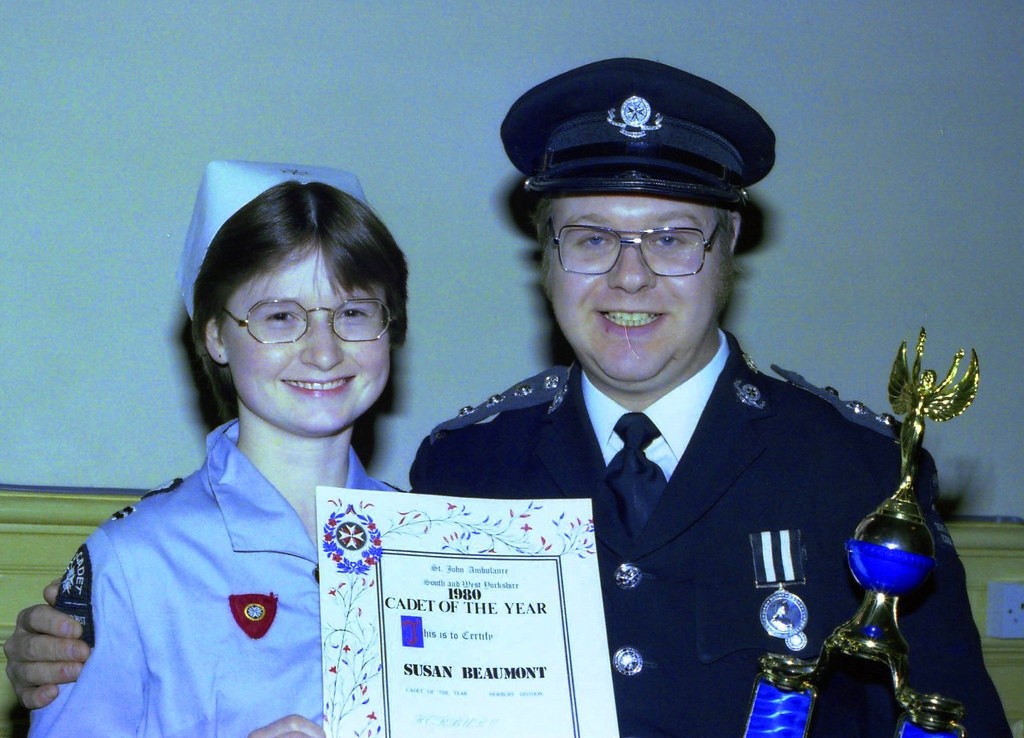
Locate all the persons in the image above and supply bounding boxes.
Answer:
[26,160,416,738]
[4,57,1013,738]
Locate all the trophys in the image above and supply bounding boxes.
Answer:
[739,328,982,738]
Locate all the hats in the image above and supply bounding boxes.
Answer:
[499,57,776,206]
[174,158,368,323]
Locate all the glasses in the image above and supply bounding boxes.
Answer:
[550,217,723,277]
[222,297,397,343]
